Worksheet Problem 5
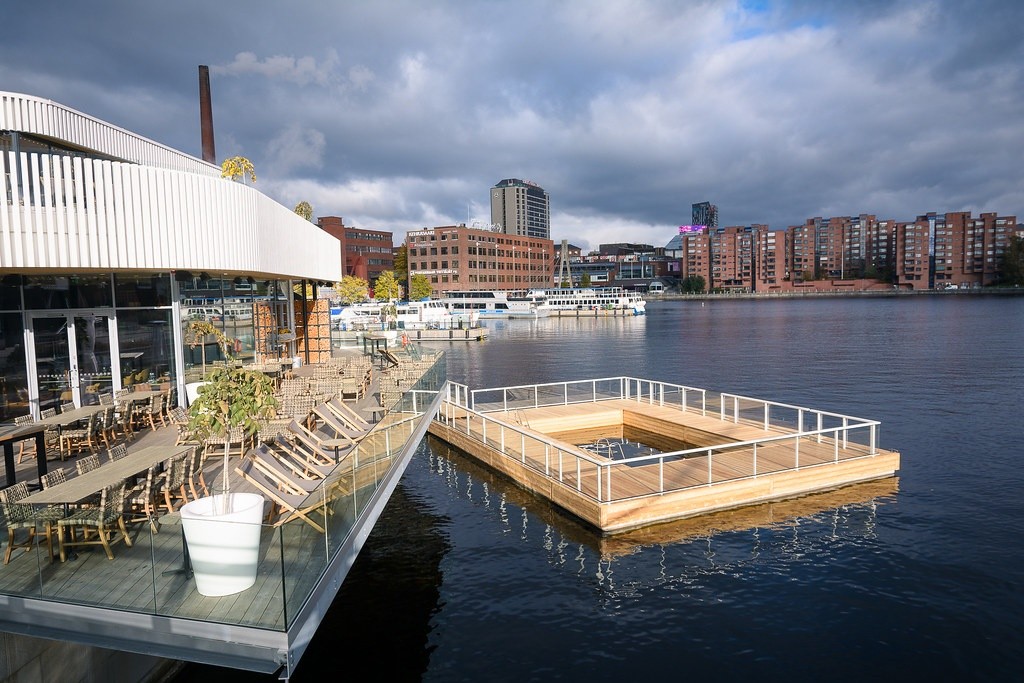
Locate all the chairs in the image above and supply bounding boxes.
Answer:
[0,349,435,563]
[260,369,282,379]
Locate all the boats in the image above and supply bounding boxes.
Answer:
[330,286,647,328]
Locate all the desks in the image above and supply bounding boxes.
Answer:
[31,405,117,461]
[364,334,388,364]
[158,511,194,580]
[362,407,385,424]
[15,446,195,562]
[308,376,357,394]
[0,426,47,503]
[114,391,166,432]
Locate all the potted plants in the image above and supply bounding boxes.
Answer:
[180,319,282,597]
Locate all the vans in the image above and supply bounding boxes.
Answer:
[944,285,958,290]
